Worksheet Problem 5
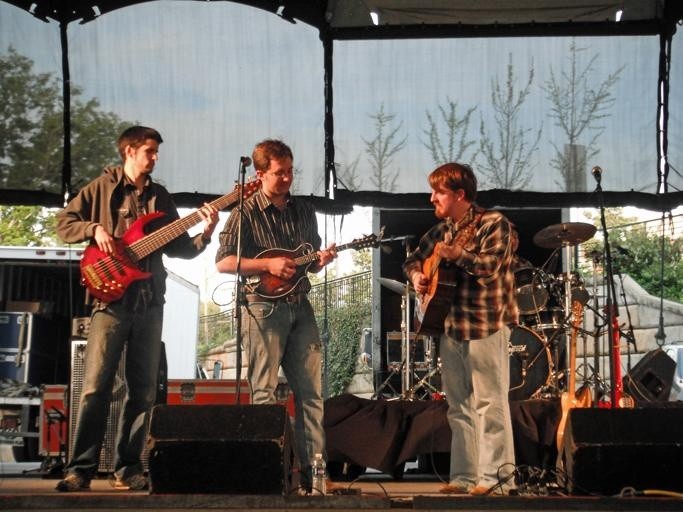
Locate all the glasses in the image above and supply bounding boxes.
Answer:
[266,168,297,177]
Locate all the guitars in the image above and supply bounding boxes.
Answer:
[556,300,592,451]
[413,205,475,335]
[597,306,634,409]
[79,176,261,302]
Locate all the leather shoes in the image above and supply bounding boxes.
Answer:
[441,481,508,497]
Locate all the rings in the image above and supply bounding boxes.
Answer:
[280,273,287,278]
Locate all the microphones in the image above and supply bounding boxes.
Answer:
[240,155,252,165]
[591,165,602,181]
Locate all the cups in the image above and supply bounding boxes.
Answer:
[212,360,222,380]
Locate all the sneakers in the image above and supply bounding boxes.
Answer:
[108,470,148,490]
[56,472,91,492]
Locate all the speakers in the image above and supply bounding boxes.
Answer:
[147,404,311,495]
[555,407,682,495]
[623,348,677,407]
[68,338,168,477]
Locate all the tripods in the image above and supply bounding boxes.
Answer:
[371,289,446,401]
[530,240,617,402]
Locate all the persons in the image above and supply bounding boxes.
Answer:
[403,162,518,496]
[214,138,344,493]
[52,123,220,491]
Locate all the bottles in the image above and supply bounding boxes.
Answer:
[312,452,327,496]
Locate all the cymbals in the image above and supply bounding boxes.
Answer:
[376,277,416,297]
[533,222,596,248]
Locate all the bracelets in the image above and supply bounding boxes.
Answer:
[411,271,420,284]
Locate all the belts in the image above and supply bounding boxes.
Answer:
[244,292,307,303]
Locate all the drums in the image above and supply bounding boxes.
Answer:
[511,267,550,315]
[523,310,565,335]
[506,322,554,400]
[550,272,588,309]
